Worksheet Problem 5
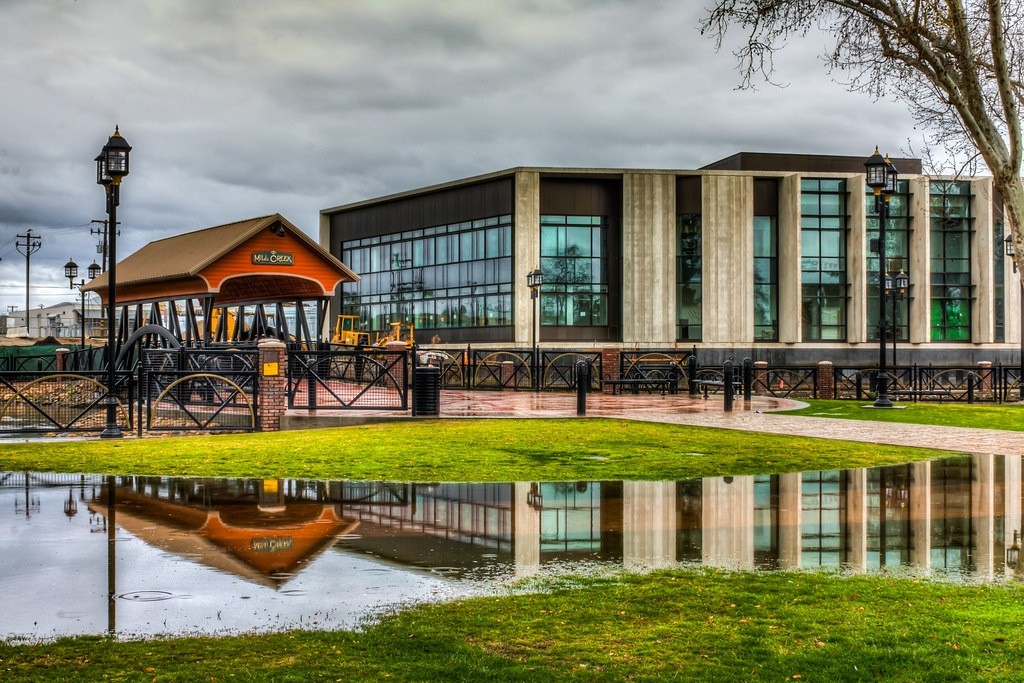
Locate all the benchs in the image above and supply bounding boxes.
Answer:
[691,375,743,400]
[224,379,302,406]
[603,371,680,395]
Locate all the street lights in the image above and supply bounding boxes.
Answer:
[886,268,911,403]
[92,123,134,438]
[862,144,894,407]
[526,264,544,390]
[63,257,102,370]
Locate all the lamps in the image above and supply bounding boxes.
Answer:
[270,220,287,237]
